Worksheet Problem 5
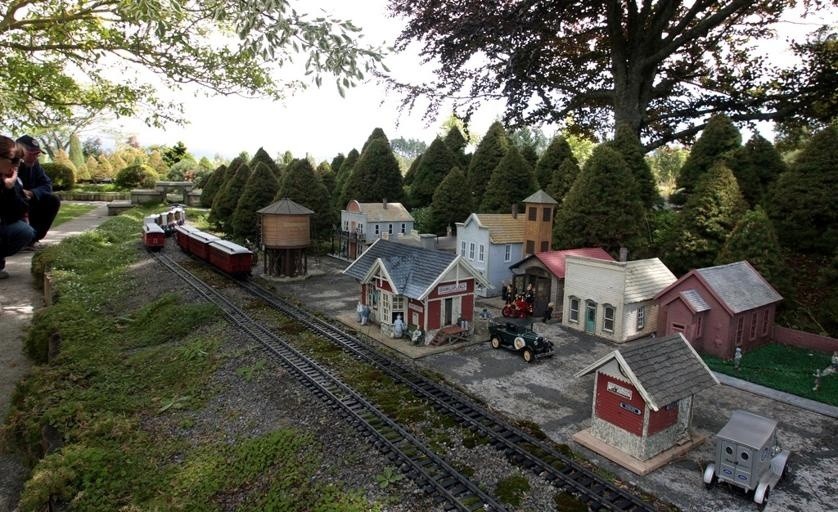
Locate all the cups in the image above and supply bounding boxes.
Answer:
[4,156,20,165]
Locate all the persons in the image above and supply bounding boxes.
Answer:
[0,135,38,281]
[11,135,62,253]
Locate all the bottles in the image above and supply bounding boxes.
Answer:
[16,134,46,154]
